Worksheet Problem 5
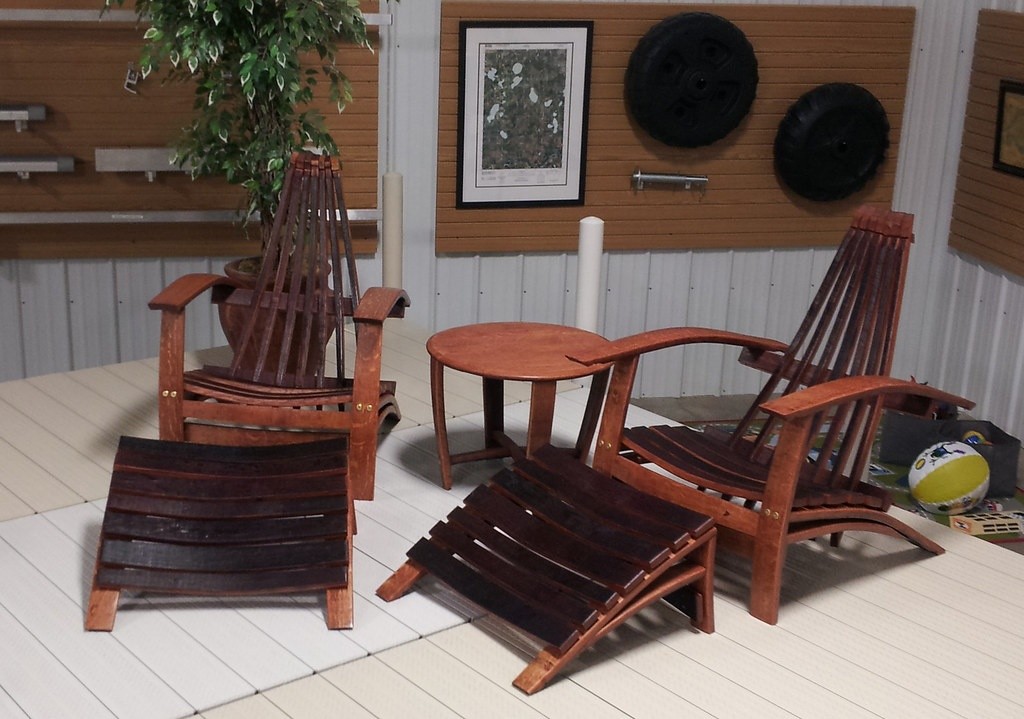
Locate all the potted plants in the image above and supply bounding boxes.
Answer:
[96,0,400,375]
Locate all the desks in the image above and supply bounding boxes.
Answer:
[425,322,616,490]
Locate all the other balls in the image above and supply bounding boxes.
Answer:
[963,431,986,444]
[910,439,993,518]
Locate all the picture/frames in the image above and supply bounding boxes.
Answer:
[990,79,1024,180]
[456,20,594,210]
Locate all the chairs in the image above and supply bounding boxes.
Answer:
[376,201,976,697]
[83,147,410,632]
